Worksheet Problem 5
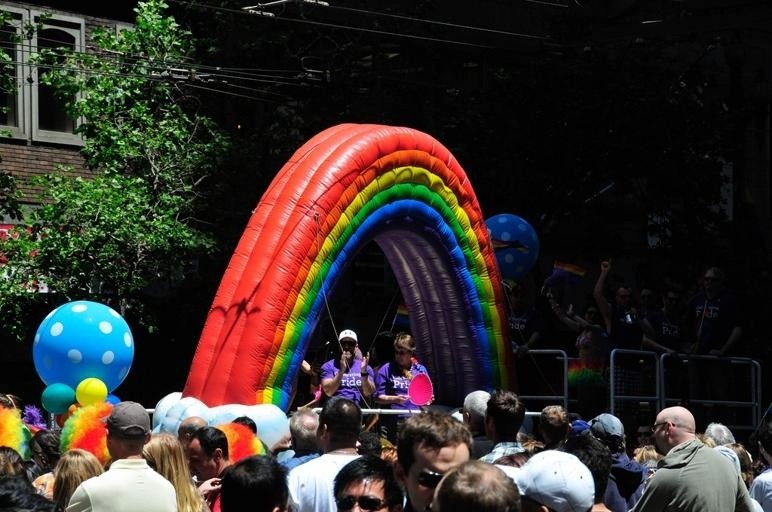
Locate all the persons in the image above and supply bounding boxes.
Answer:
[320,328,376,407]
[373,333,435,446]
[300,356,322,407]
[499,256,771,387]
[0,388,771,511]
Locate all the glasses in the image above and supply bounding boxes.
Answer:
[413,466,443,488]
[394,349,410,356]
[335,496,388,512]
[704,276,717,283]
[649,422,675,433]
[634,432,651,438]
[340,340,355,347]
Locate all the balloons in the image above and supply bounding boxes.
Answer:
[32,300,136,428]
[485,212,545,290]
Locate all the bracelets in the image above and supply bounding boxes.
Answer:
[360,371,369,376]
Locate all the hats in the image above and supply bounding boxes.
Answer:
[100,401,150,440]
[338,330,358,343]
[586,413,625,438]
[494,450,596,512]
